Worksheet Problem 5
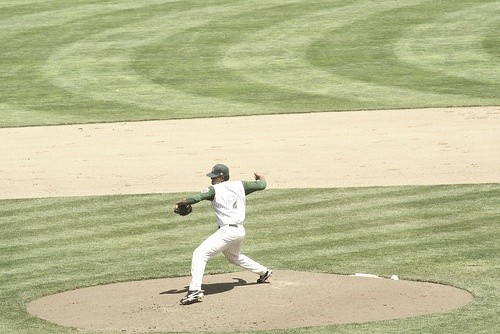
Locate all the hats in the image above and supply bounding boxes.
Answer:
[206,163,229,179]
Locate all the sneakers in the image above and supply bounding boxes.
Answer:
[180,289,204,305]
[257,268,272,284]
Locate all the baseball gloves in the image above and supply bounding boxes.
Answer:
[173,201,193,216]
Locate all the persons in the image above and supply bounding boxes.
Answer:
[175,162,274,305]
